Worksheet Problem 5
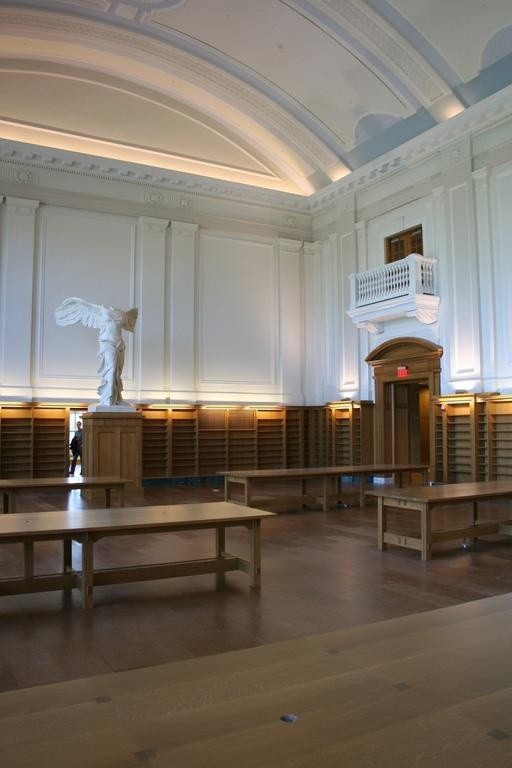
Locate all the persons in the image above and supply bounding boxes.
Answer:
[96,305,128,407]
[68,420,83,475]
